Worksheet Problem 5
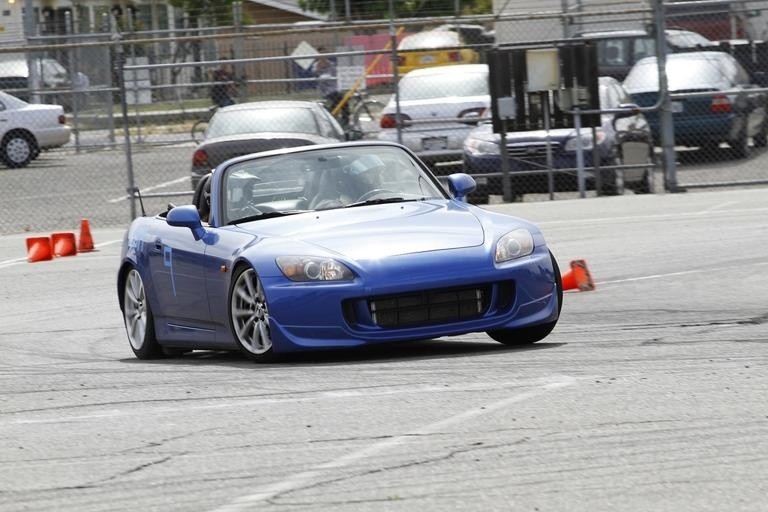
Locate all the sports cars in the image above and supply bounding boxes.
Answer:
[115,141,565,360]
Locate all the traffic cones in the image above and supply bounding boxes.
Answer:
[25,237,52,264]
[560,259,595,294]
[51,232,77,259]
[76,219,99,253]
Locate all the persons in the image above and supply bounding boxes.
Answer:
[317,153,388,208]
[203,169,266,227]
[312,47,361,141]
[209,57,241,108]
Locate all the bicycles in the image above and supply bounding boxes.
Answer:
[315,90,386,140]
[192,107,223,145]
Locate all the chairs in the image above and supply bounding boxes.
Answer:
[193,157,391,222]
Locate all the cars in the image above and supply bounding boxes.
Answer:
[190,99,364,200]
[0,59,88,112]
[0,90,72,168]
